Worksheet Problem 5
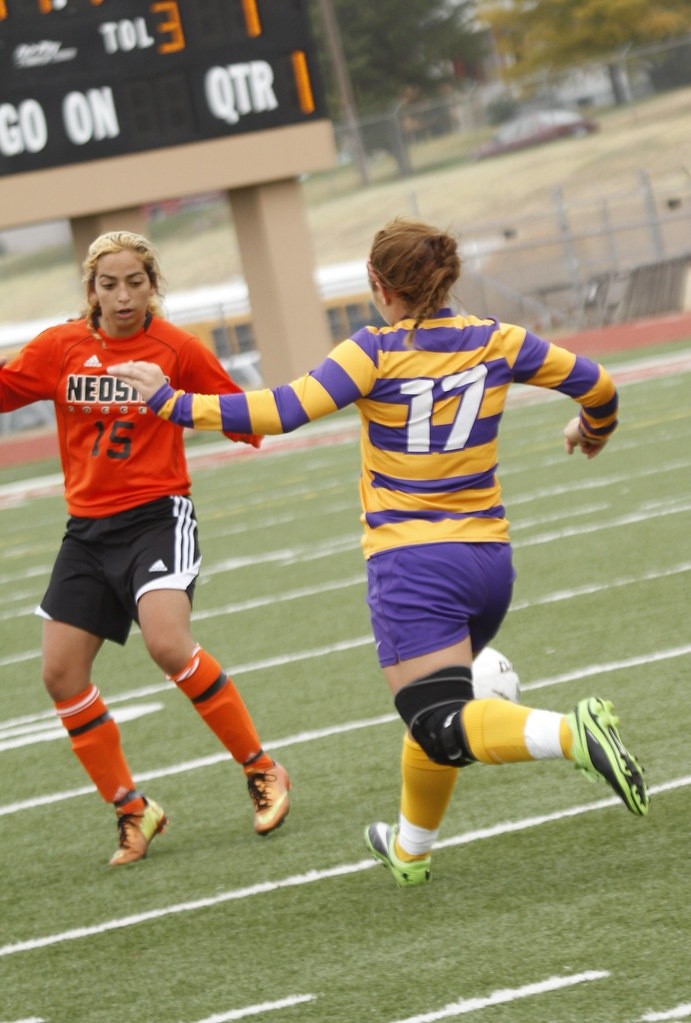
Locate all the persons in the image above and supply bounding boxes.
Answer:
[107,220,650,885]
[0,230,291,865]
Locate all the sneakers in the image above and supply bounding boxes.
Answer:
[247,759,293,836]
[566,696,649,817]
[363,820,433,886]
[109,794,168,864]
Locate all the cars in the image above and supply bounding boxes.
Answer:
[474,110,598,159]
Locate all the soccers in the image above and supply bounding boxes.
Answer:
[471,645,521,704]
